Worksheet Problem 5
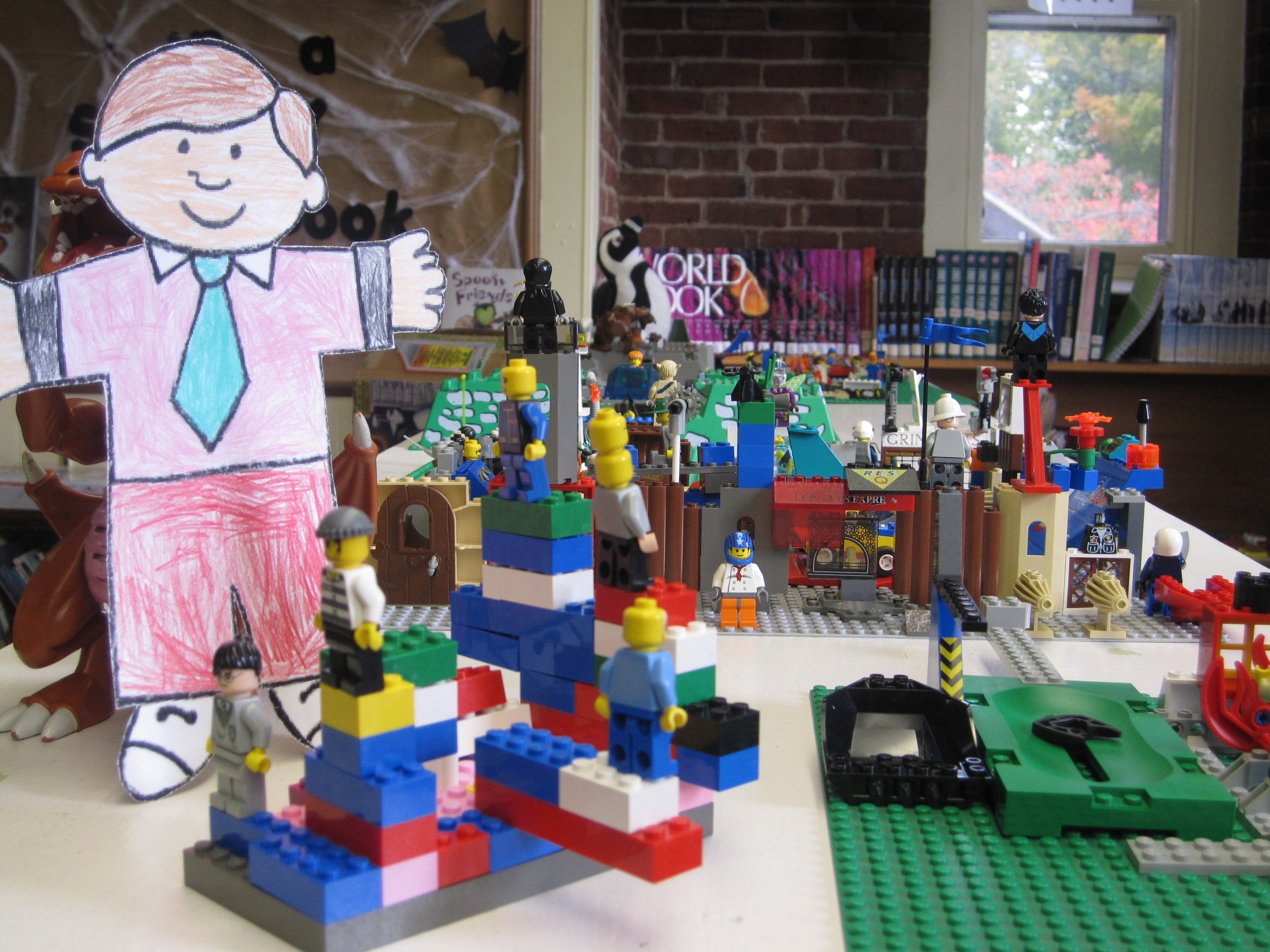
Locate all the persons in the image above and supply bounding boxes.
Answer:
[207,257,1190,819]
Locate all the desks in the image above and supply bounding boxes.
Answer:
[0,367,1270,952]
[887,357,1270,541]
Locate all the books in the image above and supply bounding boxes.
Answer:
[643,246,1270,362]
